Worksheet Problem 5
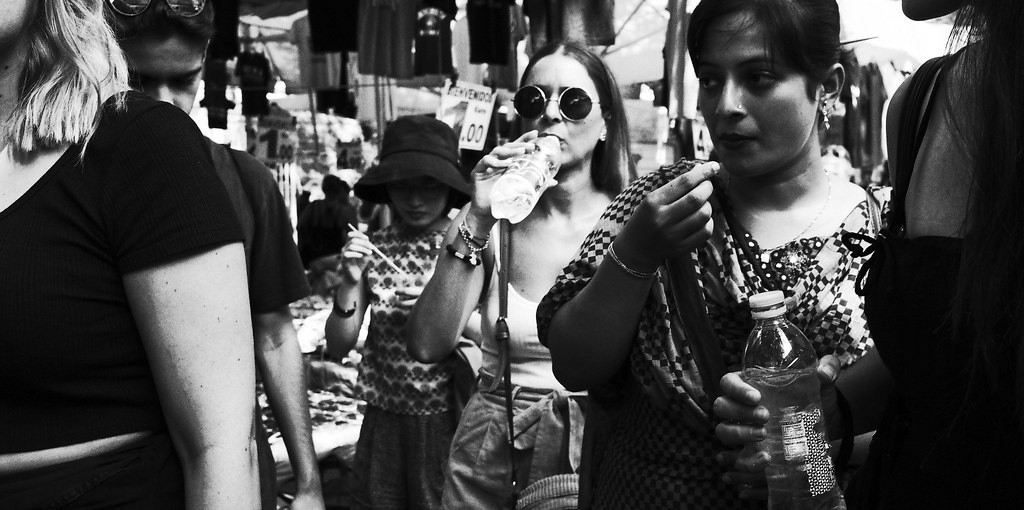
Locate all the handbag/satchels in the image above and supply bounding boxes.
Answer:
[514,473,579,510]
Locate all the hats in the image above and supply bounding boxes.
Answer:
[352,114,474,210]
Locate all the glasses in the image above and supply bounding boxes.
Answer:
[109,0,207,17]
[510,85,601,122]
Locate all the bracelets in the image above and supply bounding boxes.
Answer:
[458,219,490,266]
[605,241,660,280]
[334,295,357,319]
[465,235,483,248]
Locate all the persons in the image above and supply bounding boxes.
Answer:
[445,243,482,266]
[297,0,1024,510]
[99,0,332,510]
[461,222,486,248]
[0,0,271,510]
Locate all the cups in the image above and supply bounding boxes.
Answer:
[392,274,423,300]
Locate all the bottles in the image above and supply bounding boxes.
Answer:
[490,136,562,224]
[740,290,847,510]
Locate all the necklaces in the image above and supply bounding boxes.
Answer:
[720,170,833,251]
